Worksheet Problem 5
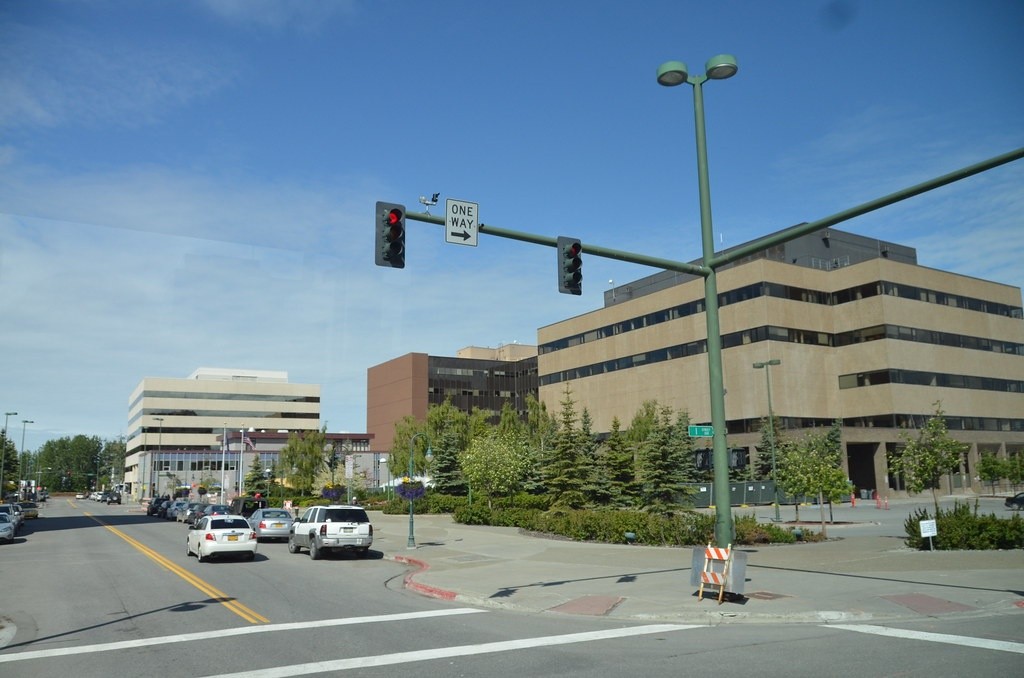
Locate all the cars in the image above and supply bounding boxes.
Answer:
[246,508,295,543]
[186,514,258,562]
[0,491,50,544]
[147,497,236,526]
[1004,491,1024,511]
[89,490,112,503]
[76,492,85,499]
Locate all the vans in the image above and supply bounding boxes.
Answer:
[227,497,268,520]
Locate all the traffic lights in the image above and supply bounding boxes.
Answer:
[376,201,406,269]
[556,235,583,296]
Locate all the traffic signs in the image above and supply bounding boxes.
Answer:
[445,198,479,247]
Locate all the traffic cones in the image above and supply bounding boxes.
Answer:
[882,495,891,510]
[876,494,882,508]
[849,492,857,508]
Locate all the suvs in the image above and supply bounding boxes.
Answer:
[106,492,121,504]
[287,505,374,560]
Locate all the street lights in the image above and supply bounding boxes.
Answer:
[17,419,36,501]
[375,454,388,495]
[264,460,275,495]
[405,433,435,547]
[328,443,351,505]
[0,412,19,500]
[752,358,782,523]
[38,466,52,487]
[656,54,739,554]
[152,417,165,493]
